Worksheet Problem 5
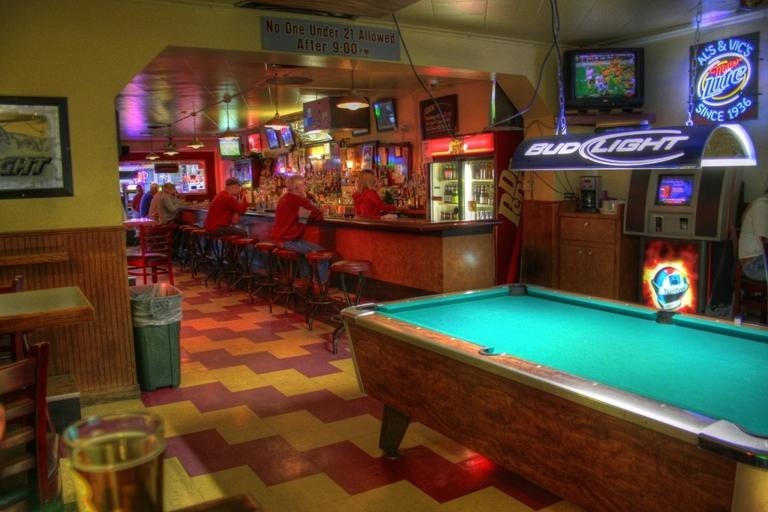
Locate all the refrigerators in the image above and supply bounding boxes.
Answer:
[426,132,525,287]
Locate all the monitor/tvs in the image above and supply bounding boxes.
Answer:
[217,124,296,160]
[564,47,645,115]
[655,174,693,206]
[373,99,398,132]
[490,77,525,132]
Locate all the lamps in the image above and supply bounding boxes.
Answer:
[509,0,757,172]
[217,99,240,142]
[144,132,160,161]
[162,125,180,156]
[185,112,206,149]
[264,73,291,130]
[336,60,370,112]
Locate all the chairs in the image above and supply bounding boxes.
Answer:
[127,220,175,286]
[0,275,65,512]
[760,236,768,283]
[729,224,768,324]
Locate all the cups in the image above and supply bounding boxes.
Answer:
[62,408,169,512]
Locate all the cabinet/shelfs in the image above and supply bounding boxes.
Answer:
[558,199,640,303]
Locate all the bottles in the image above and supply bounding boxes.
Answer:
[436,161,495,220]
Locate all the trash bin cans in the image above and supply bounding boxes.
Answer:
[129,283,183,391]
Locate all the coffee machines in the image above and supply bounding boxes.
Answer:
[576,175,602,213]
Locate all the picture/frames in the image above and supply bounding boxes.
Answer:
[0,95,74,199]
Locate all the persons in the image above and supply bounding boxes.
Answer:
[351,169,392,222]
[203,177,248,261]
[271,175,329,294]
[738,187,768,281]
[132,183,186,261]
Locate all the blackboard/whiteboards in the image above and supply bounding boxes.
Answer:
[419,93,458,141]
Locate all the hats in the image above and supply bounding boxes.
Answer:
[226,177,242,187]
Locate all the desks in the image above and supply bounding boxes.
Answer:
[0,285,95,428]
[338,282,767,512]
[0,251,70,266]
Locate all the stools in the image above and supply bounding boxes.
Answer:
[176,224,373,355]
